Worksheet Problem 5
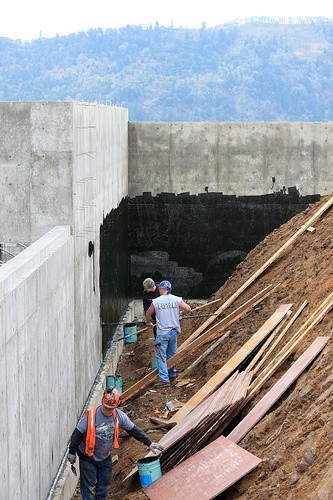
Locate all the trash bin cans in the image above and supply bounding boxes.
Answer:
[124,323,137,342]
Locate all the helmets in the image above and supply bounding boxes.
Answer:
[143,278,155,289]
[158,280,173,290]
[102,388,121,408]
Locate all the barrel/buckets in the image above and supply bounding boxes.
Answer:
[137,456,163,488]
[106,376,123,394]
[125,323,137,343]
[151,357,158,370]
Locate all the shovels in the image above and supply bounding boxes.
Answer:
[68,464,77,476]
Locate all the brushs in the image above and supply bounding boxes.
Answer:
[106,298,221,349]
[100,315,208,326]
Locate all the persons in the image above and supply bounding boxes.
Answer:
[146,280,191,388]
[67,388,164,500]
[142,278,160,340]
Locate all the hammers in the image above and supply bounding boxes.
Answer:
[155,343,165,362]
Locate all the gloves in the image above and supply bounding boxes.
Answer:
[67,453,76,465]
[148,441,163,454]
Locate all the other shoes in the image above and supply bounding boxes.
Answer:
[154,382,170,387]
[169,377,177,380]
